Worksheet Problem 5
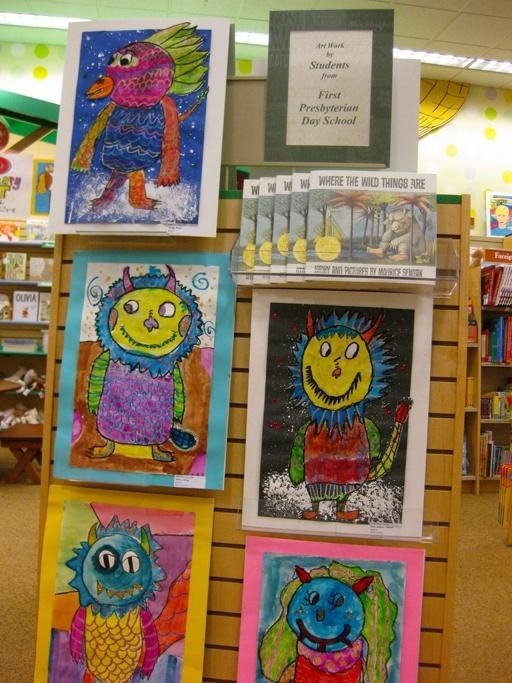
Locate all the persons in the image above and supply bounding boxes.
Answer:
[491,208,512,237]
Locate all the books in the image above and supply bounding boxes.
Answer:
[481,260,512,477]
[0,221,52,352]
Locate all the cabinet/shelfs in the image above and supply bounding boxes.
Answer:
[460,248,511,495]
[0,235,56,430]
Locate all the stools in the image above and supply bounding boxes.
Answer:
[0,423,43,485]
